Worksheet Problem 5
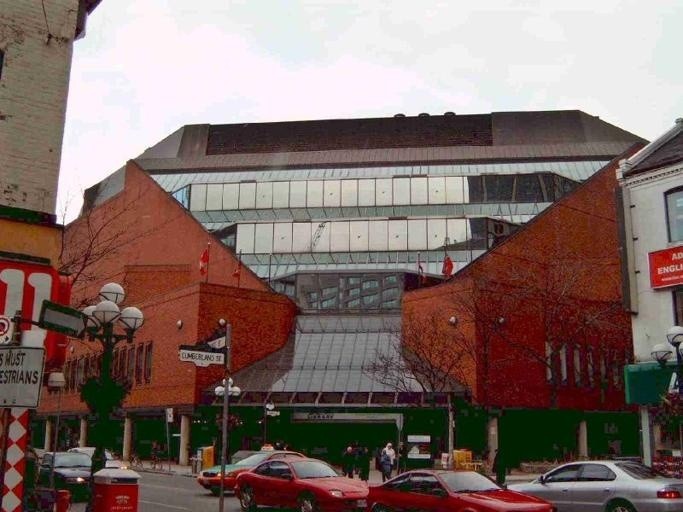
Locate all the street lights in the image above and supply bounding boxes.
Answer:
[650,325,683,398]
[81,282,144,508]
[214,376,241,460]
[261,395,275,452]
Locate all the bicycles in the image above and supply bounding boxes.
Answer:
[129,451,142,468]
[148,451,163,471]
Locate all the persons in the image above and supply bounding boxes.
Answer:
[342,437,511,487]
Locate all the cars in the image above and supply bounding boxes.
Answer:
[506,459,683,511]
[197,443,309,495]
[365,468,554,512]
[233,457,369,512]
[36,451,92,493]
[67,447,127,471]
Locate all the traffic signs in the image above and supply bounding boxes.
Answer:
[205,332,226,348]
[178,344,226,367]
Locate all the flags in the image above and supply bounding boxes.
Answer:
[232,249,241,276]
[197,248,208,276]
[440,252,454,275]
[418,252,423,274]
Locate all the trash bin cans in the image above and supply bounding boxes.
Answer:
[91,469,143,512]
